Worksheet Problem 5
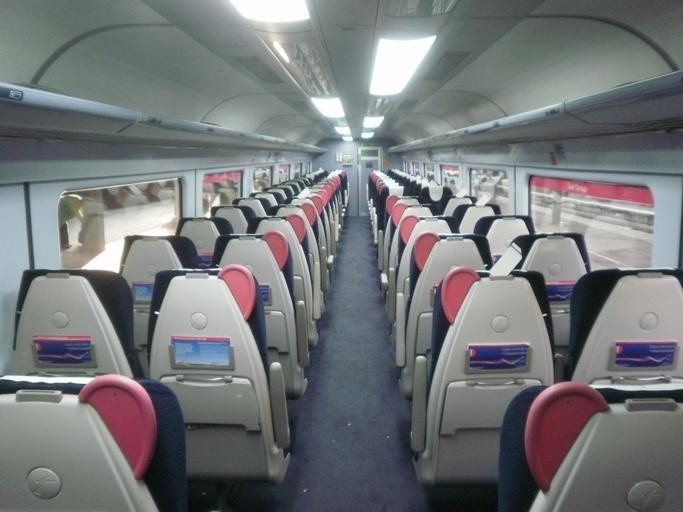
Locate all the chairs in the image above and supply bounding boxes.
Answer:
[367,167,435,186]
[367,186,377,244]
[420,186,457,215]
[381,204,444,295]
[443,195,480,222]
[177,217,234,269]
[248,215,321,354]
[265,203,330,295]
[253,168,348,199]
[147,266,291,483]
[498,382,683,512]
[506,230,593,348]
[386,215,461,326]
[229,195,268,220]
[11,269,143,383]
[453,202,500,235]
[408,268,555,491]
[0,373,190,512]
[555,265,682,384]
[277,199,334,294]
[210,231,307,397]
[472,212,535,269]
[119,233,202,381]
[393,233,493,399]
[208,204,256,236]
[335,198,347,256]
